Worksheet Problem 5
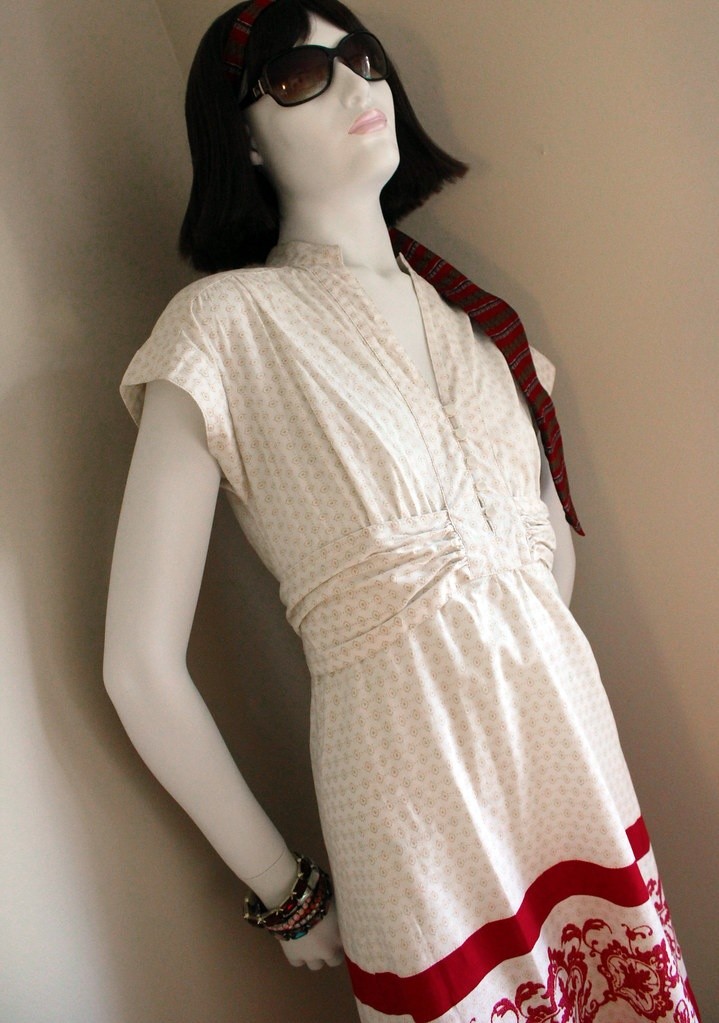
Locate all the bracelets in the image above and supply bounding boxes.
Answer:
[243,855,334,939]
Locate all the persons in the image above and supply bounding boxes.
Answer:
[94,0,701,1022]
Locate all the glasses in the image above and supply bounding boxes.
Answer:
[237,30,390,112]
